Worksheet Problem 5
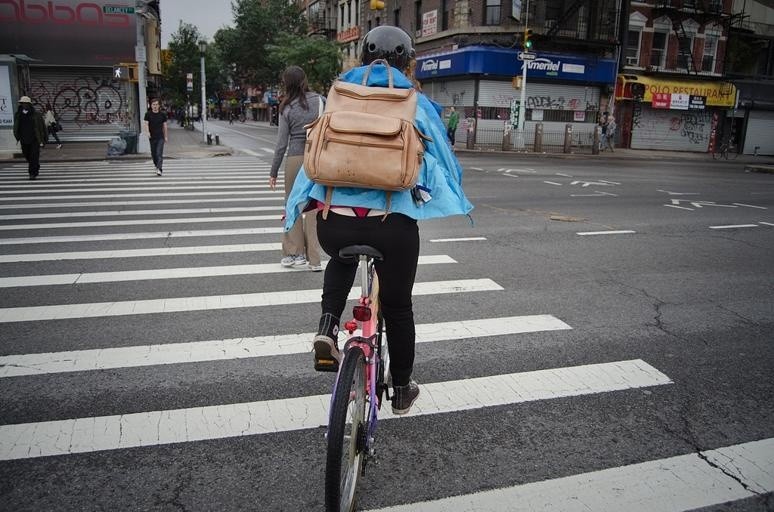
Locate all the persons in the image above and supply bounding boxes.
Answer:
[446,106,459,152]
[269,64,327,272]
[599,115,617,152]
[599,116,608,149]
[144,99,168,177]
[40,102,63,151]
[12,94,45,181]
[164,105,186,128]
[228,109,233,125]
[282,24,475,416]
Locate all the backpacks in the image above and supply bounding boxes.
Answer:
[303,59,433,190]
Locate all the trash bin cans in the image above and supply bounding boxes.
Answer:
[119,130,137,154]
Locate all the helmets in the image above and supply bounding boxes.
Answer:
[357,26,416,73]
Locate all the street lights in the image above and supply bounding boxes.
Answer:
[198,39,207,142]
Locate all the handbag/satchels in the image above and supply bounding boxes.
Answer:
[51,121,62,132]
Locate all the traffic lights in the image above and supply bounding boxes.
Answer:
[112,65,129,80]
[370,0,385,11]
[523,27,535,50]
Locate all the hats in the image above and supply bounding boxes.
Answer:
[17,96,32,104]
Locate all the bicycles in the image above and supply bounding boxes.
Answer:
[599,133,614,151]
[712,134,739,160]
[325,244,394,510]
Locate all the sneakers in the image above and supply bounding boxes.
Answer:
[40,142,44,146]
[153,168,162,177]
[392,379,420,415]
[281,255,323,272]
[29,171,39,180]
[312,312,342,373]
[56,145,62,148]
